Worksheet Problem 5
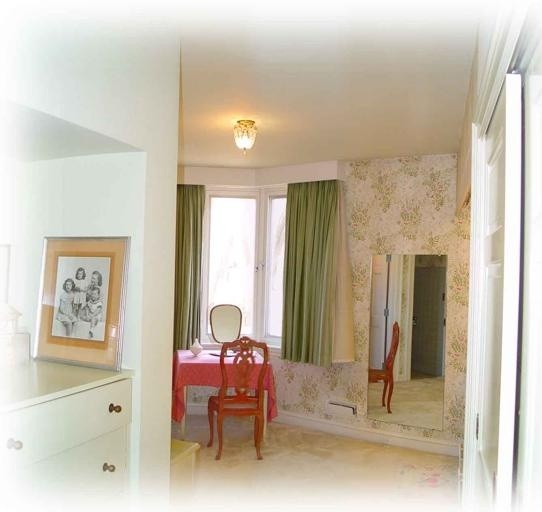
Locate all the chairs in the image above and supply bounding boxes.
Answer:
[368,321,399,413]
[206,337,268,463]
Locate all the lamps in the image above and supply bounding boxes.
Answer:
[233,121,258,152]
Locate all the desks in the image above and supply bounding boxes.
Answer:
[174,348,267,441]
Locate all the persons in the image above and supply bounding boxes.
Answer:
[81,287,102,337]
[72,267,86,317]
[56,278,78,337]
[88,271,103,292]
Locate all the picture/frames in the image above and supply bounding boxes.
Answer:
[33,235,132,372]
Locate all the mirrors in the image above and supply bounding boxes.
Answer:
[211,304,242,356]
[366,254,447,432]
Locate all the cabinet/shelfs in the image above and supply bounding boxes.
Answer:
[171,438,200,500]
[9,358,134,480]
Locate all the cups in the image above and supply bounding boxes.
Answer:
[190,346,204,358]
